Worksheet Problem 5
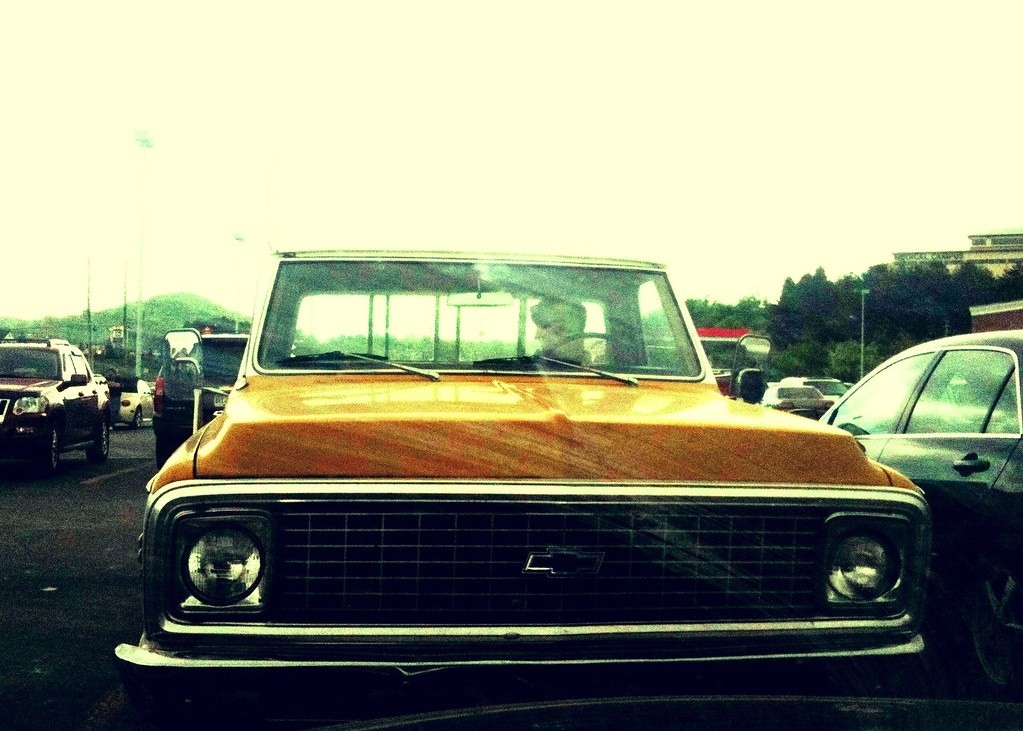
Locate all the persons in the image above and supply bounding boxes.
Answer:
[532,299,635,364]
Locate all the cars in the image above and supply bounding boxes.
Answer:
[107,373,155,429]
[116,249,1023,731]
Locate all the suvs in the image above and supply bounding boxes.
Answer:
[0,336,112,473]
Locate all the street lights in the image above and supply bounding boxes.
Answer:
[853,287,871,379]
